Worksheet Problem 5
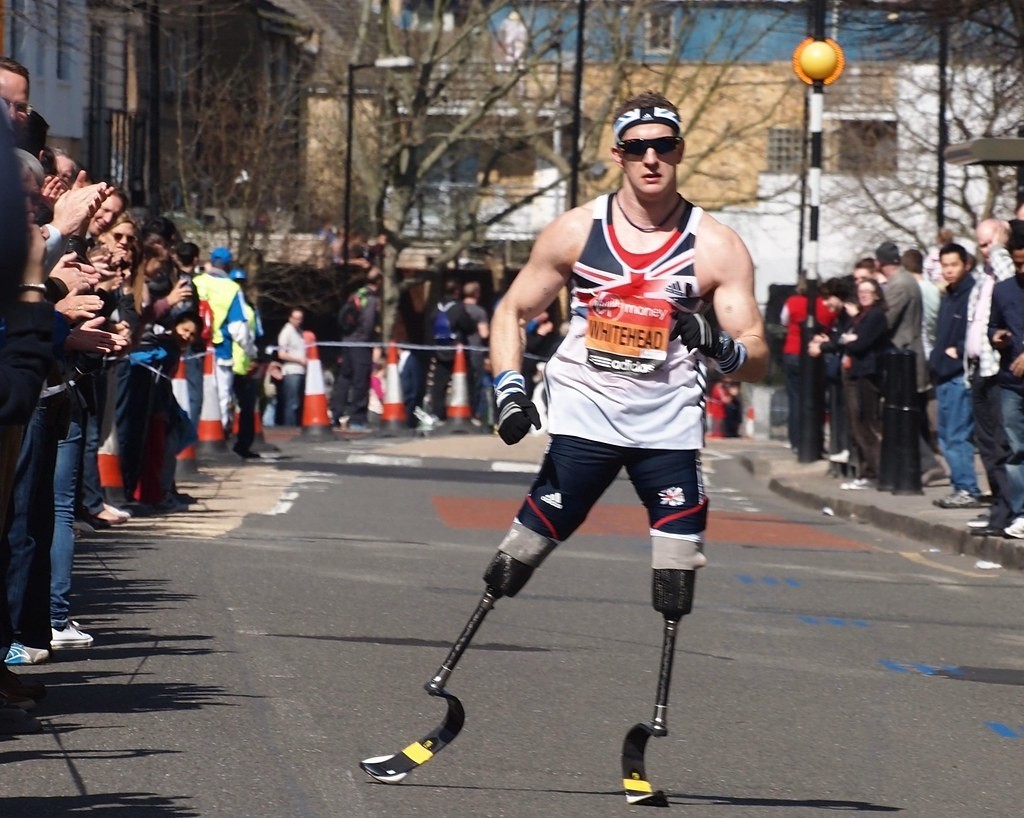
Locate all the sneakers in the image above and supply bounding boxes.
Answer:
[3,643,51,666]
[50,621,95,650]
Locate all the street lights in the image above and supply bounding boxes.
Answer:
[797,42,838,280]
[340,52,417,332]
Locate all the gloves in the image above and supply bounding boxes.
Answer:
[669,309,724,359]
[495,392,542,445]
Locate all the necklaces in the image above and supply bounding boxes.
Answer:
[615,187,682,232]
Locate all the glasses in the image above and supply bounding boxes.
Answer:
[617,135,681,156]
[109,230,136,243]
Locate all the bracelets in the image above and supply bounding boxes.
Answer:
[19,282,46,294]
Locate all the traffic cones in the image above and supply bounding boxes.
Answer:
[291,328,346,444]
[743,403,756,440]
[227,390,278,452]
[434,345,484,437]
[96,413,142,519]
[703,400,716,437]
[190,345,241,468]
[371,339,425,438]
[170,353,207,482]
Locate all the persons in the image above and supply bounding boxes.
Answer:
[0,56,261,663]
[267,270,568,435]
[780,202,1024,537]
[358,91,768,805]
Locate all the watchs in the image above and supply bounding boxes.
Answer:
[718,331,735,362]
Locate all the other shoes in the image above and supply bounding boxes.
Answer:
[74,503,130,539]
[828,449,850,464]
[966,514,1007,537]
[239,449,260,459]
[1002,517,1024,539]
[926,471,952,487]
[837,475,871,491]
[0,673,46,734]
[931,490,981,509]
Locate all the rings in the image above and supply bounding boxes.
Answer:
[78,304,83,310]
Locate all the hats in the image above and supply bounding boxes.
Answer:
[875,241,899,264]
[209,247,232,267]
[229,268,247,281]
